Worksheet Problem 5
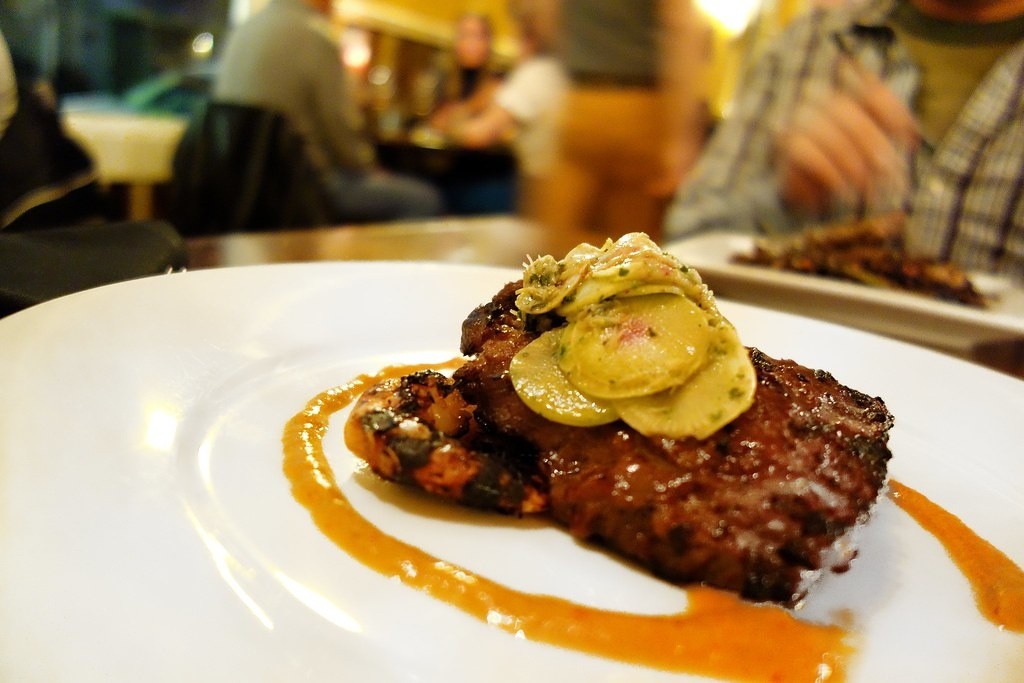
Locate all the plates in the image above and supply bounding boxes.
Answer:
[0,256,1024,683]
[655,229,1023,356]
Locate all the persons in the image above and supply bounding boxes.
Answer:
[659,0,1024,286]
[551,0,693,248]
[0,32,104,231]
[417,10,592,229]
[218,0,439,230]
